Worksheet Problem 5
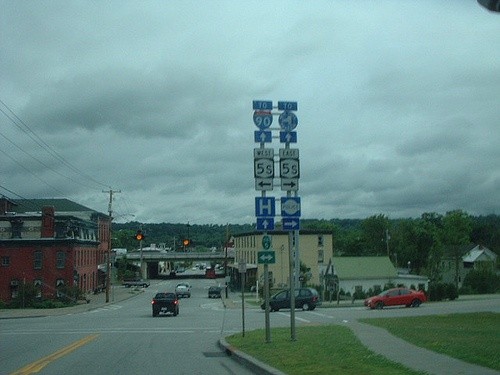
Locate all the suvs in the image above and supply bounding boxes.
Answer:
[260,287,321,312]
[122,277,151,288]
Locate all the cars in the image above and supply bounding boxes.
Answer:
[169,262,206,276]
[208,285,222,299]
[364,287,427,311]
[176,281,193,299]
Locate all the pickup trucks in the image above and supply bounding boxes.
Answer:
[151,290,180,317]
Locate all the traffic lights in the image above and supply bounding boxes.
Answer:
[136,233,143,240]
[183,239,189,246]
